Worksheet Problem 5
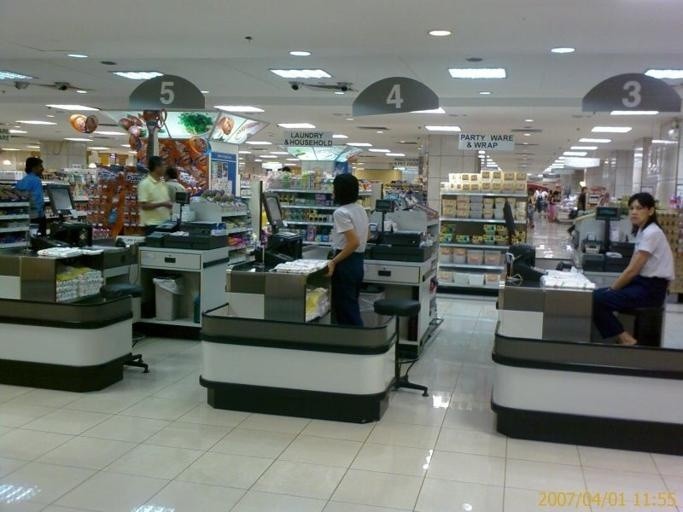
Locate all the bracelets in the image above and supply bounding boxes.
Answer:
[331,259,337,265]
[608,286,616,291]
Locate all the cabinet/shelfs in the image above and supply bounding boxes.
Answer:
[0,144,683,291]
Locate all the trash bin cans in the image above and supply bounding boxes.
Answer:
[155,273,184,321]
[359,287,386,328]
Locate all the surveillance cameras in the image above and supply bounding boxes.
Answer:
[56,82,69,90]
[15,82,28,89]
[338,83,351,91]
[289,82,303,90]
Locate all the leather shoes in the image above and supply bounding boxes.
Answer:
[634,340,640,345]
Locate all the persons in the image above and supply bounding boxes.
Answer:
[137,154,175,236]
[590,191,676,346]
[12,155,48,236]
[319,172,372,327]
[525,182,611,236]
[163,166,190,206]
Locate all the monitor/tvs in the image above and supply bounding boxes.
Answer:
[503,200,515,234]
[47,185,76,214]
[262,193,283,226]
